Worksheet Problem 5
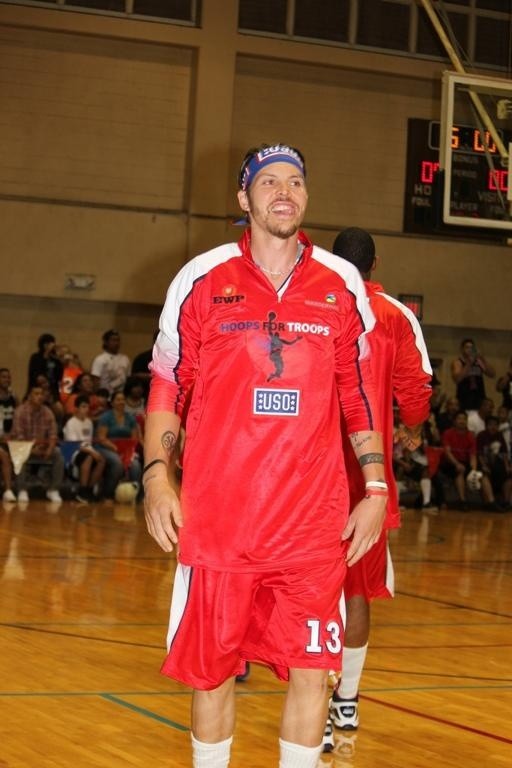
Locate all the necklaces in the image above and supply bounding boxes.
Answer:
[250,238,302,276]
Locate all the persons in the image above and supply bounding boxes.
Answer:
[392,338,511,511]
[321,224,433,754]
[135,142,390,768]
[1,325,159,501]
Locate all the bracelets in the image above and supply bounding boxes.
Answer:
[365,481,389,491]
[138,459,170,483]
[364,489,388,497]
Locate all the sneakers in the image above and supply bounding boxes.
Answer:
[322,678,359,754]
[3,490,100,503]
